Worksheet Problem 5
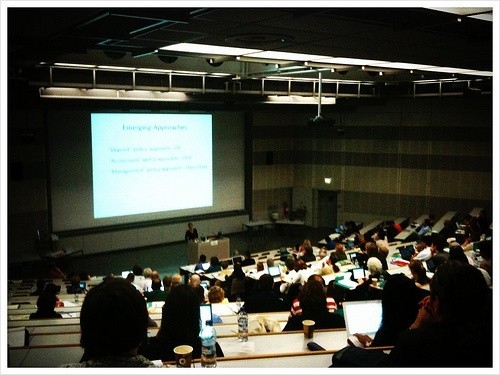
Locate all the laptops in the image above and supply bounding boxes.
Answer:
[341,299,384,350]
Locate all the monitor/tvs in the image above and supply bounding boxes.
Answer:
[121,271,133,280]
[80,282,86,289]
[200,305,213,327]
[340,224,345,231]
[349,252,358,261]
[335,228,340,233]
[200,283,207,288]
[352,268,365,280]
[268,266,280,277]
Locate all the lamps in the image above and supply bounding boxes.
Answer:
[324,177,332,184]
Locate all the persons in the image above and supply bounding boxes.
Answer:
[136,282,225,360]
[333,262,493,367]
[224,206,493,298]
[353,271,433,347]
[283,280,347,333]
[186,223,200,266]
[206,256,223,275]
[237,273,289,314]
[28,292,66,320]
[289,273,337,321]
[195,255,212,274]
[203,285,237,316]
[59,277,162,368]
[31,263,208,307]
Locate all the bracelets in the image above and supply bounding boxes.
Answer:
[412,322,427,331]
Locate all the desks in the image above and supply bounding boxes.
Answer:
[7,207,493,369]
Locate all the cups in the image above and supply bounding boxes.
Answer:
[302,320,315,338]
[372,278,378,286]
[173,345,193,367]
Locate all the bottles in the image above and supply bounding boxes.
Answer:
[236,307,249,343]
[197,319,218,368]
[379,274,385,288]
